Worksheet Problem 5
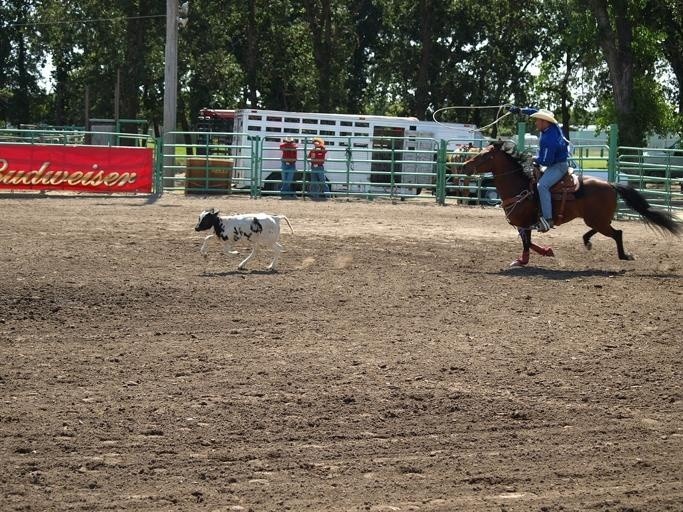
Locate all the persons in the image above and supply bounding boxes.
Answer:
[279,137,298,198]
[508,104,569,233]
[306,137,332,199]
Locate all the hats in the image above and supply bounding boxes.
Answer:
[283,136,294,143]
[530,108,558,124]
[313,136,324,144]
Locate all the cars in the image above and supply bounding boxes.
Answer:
[644,136,683,180]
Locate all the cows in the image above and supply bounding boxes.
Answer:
[194,209,294,272]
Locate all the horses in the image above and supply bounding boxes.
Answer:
[448,143,481,206]
[461,137,683,267]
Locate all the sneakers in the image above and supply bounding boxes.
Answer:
[537,218,554,233]
